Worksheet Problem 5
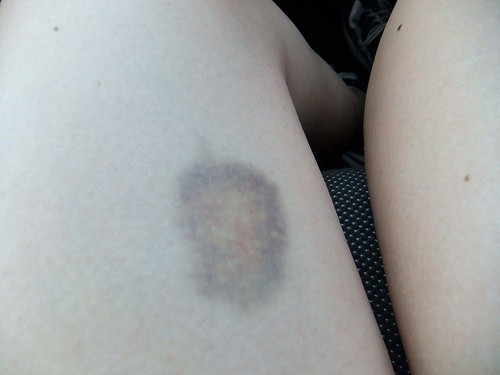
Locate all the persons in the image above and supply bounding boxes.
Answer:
[0,2,498,375]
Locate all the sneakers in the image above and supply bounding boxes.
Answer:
[340,1,395,80]
[331,46,371,167]
